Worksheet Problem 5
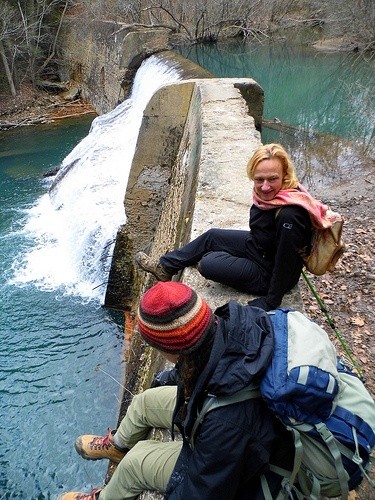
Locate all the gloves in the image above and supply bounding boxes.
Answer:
[151,368,174,388]
[247,296,279,311]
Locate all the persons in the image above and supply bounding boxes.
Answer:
[134,144,314,312]
[60,280,298,500]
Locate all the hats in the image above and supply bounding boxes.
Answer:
[137,280,214,355]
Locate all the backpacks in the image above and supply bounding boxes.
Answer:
[189,308,375,499]
[274,203,344,276]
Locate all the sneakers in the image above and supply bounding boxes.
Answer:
[136,252,173,283]
[74,429,129,465]
[61,488,102,500]
